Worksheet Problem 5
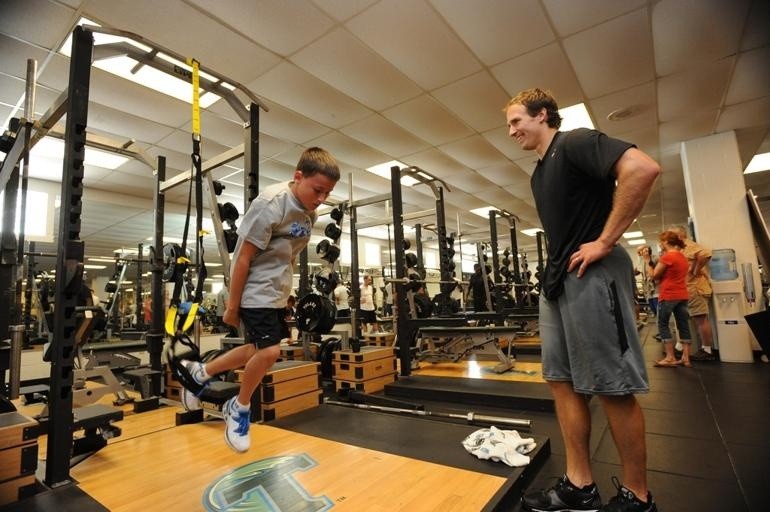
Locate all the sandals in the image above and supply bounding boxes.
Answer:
[653,358,678,368]
[678,358,693,368]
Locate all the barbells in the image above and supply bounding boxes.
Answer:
[399,229,542,318]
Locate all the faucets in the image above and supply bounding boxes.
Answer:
[729,296,735,302]
[721,298,727,303]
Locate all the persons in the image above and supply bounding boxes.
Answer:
[284,294,295,321]
[499,87,662,512]
[358,274,379,334]
[382,279,393,316]
[464,263,489,312]
[180,145,341,449]
[334,279,352,317]
[216,283,228,328]
[632,224,715,369]
[178,295,205,315]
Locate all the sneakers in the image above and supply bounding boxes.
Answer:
[598,476,658,512]
[178,358,208,411]
[521,473,601,511]
[222,395,252,453]
[690,349,719,362]
[662,349,684,357]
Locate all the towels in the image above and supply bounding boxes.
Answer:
[462,425,537,467]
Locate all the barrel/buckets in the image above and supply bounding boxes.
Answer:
[708,248,739,282]
